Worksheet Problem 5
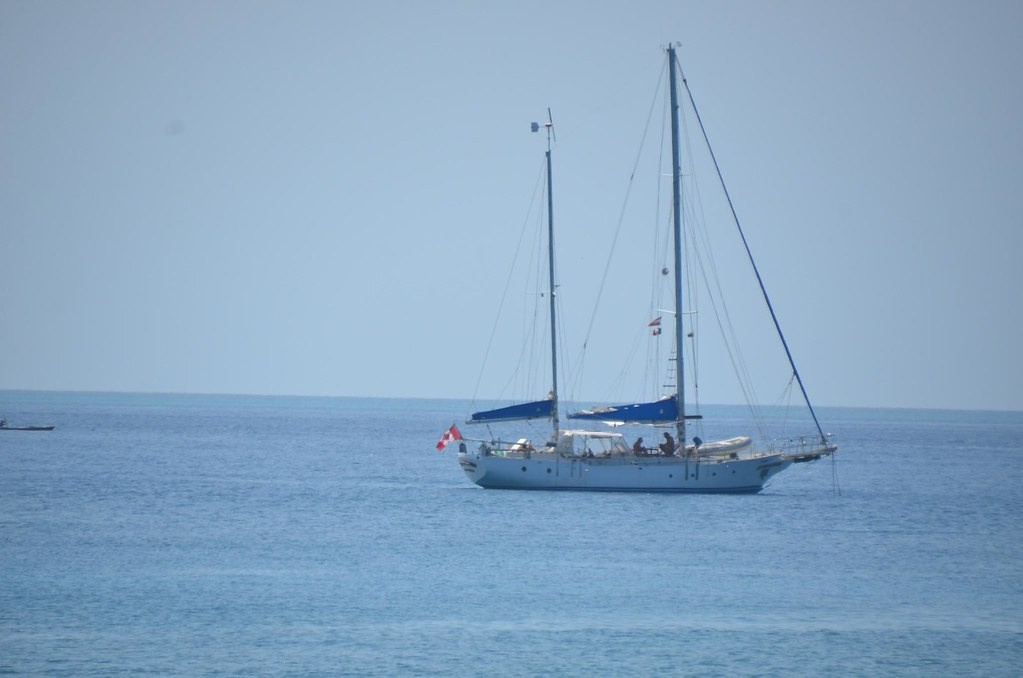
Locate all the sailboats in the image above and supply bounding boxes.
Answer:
[455,42,839,494]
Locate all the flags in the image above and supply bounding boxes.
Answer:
[436,423,461,451]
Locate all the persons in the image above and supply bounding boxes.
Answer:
[633,437,647,455]
[659,432,675,455]
